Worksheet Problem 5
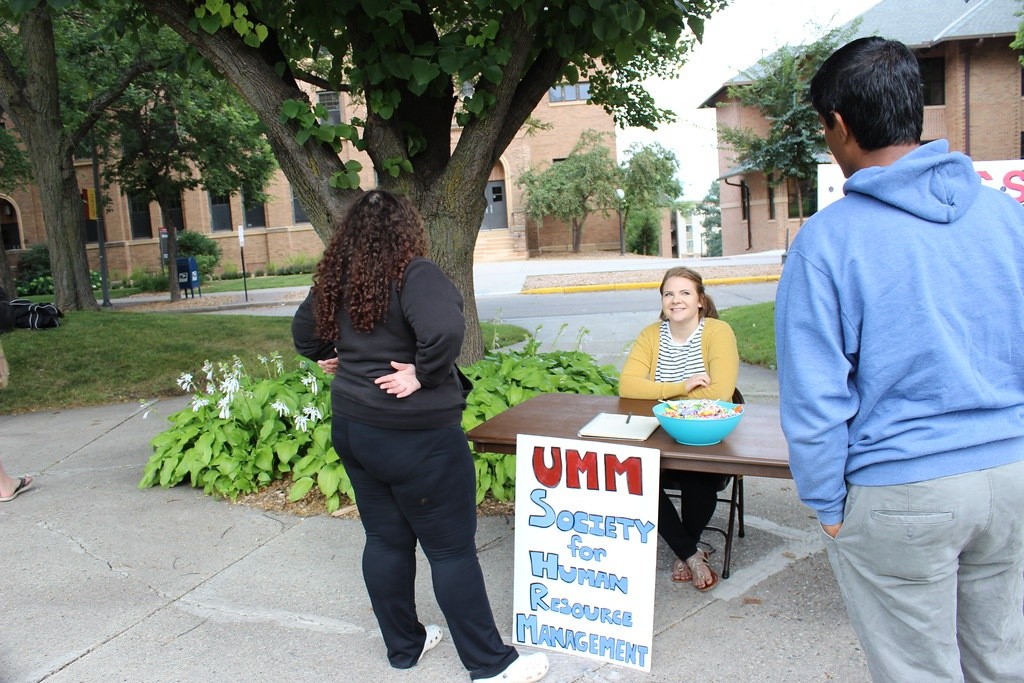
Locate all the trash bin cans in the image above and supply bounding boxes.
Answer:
[175,256,201,299]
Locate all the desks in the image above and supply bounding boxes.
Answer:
[463,393,795,480]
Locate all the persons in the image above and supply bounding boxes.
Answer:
[617,266,739,590]
[0,343,35,501]
[774,33,1024,683]
[295,197,548,682]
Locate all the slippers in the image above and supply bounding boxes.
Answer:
[0,474,33,501]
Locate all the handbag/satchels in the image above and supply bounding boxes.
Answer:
[9,299,64,330]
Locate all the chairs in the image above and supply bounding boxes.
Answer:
[665,388,745,579]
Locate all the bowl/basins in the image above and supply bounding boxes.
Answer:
[653,400,744,446]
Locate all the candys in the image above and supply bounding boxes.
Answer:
[656,397,744,420]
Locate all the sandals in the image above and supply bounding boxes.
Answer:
[472,652,549,683]
[689,548,719,592]
[416,625,443,664]
[670,554,692,583]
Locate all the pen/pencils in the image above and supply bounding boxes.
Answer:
[626,411,633,424]
